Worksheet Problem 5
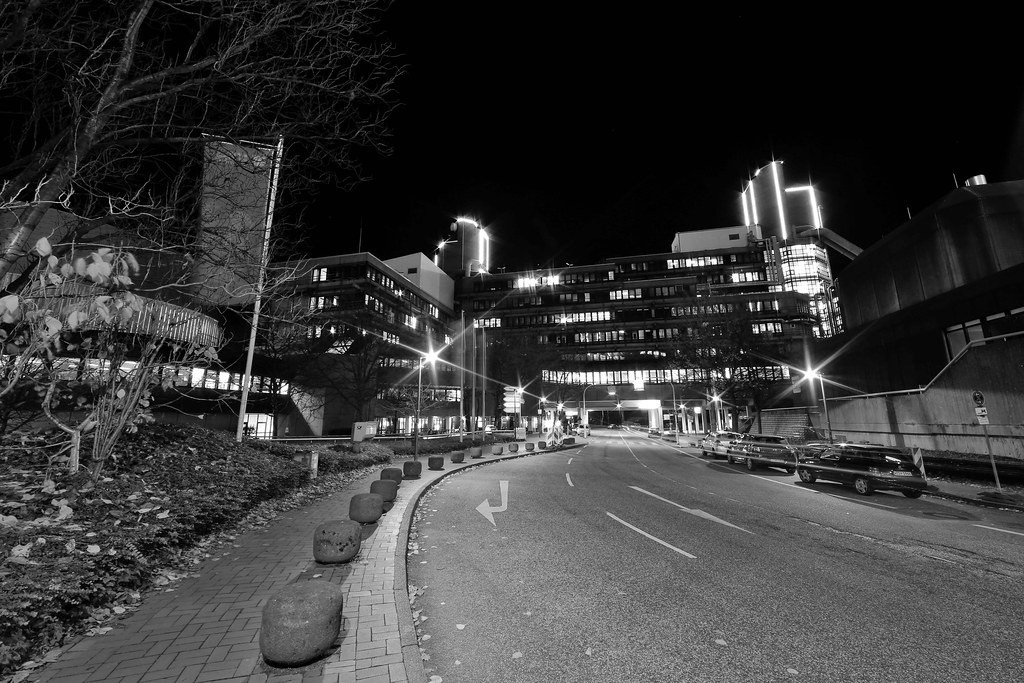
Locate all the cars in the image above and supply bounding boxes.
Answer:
[484,424,498,435]
[571,423,580,433]
[727,433,798,474]
[607,423,617,429]
[701,430,744,460]
[648,427,662,439]
[796,442,923,499]
[577,424,591,437]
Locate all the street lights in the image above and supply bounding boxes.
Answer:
[538,398,546,438]
[633,377,678,445]
[514,388,523,443]
[583,382,616,439]
[551,402,563,441]
[805,368,834,444]
[413,354,437,460]
[713,396,724,430]
[679,405,689,437]
